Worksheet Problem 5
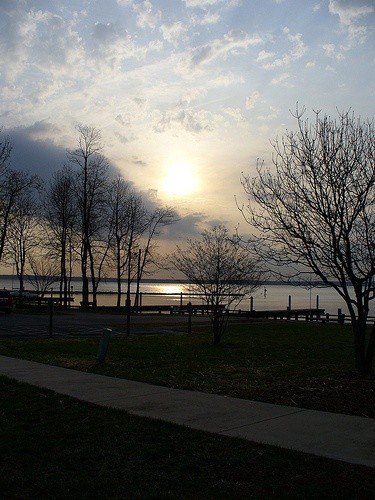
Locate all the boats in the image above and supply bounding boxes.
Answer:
[11,290,38,297]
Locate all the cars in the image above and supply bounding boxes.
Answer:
[0,290,13,314]
